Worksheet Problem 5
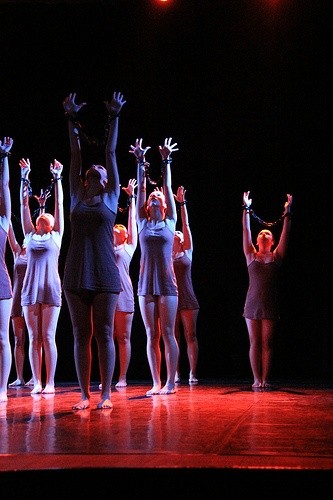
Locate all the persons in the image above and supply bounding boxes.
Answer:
[242,190,292,388]
[0,137,64,402]
[129,137,179,395]
[154,186,200,383]
[63,90,127,410]
[99,178,138,390]
